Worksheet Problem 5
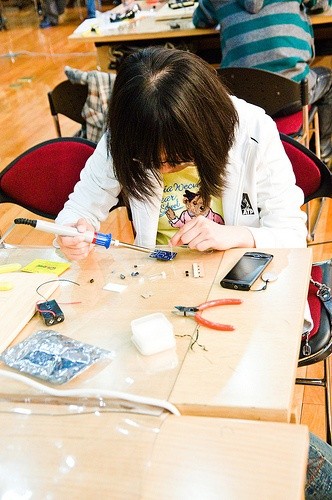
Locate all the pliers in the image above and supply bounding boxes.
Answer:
[172,298,245,332]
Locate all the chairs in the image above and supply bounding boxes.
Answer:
[48,78,88,138]
[215,66,326,243]
[279,131,332,446]
[0,136,136,240]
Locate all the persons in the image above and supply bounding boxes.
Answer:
[193,0,332,158]
[42,0,67,24]
[53,48,332,500]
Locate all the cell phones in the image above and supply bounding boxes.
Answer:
[221,252,273,291]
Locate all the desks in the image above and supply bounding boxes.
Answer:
[0,245,312,421]
[68,7,332,72]
[0,401,312,500]
